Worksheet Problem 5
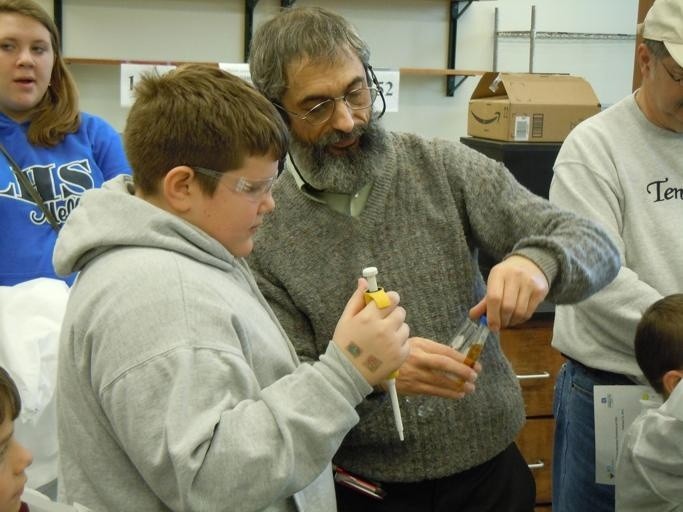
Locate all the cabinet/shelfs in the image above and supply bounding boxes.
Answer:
[460,137,564,202]
[478,298,570,512]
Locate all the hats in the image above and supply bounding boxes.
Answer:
[637,0,683,67]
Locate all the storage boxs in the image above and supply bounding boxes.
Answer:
[466,71,603,143]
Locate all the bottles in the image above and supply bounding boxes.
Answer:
[448,316,478,352]
[450,314,491,384]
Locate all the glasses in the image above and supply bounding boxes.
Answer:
[653,56,683,83]
[194,167,277,202]
[273,85,380,127]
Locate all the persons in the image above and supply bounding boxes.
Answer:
[52,61,410,511]
[0,367,49,512]
[0,0,132,501]
[244,6,620,512]
[549,0,683,512]
[614,294,683,512]
